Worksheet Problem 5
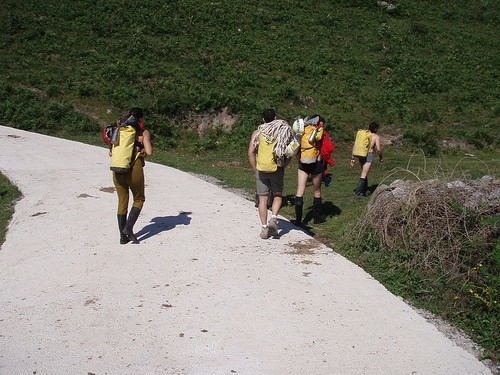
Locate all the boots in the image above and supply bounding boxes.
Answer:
[356,178,366,195]
[122,207,139,244]
[313,197,326,224]
[365,176,372,192]
[291,197,303,227]
[117,214,130,243]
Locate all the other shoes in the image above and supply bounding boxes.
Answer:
[260,225,270,239]
[268,219,281,239]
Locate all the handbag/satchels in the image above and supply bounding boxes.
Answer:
[122,113,138,127]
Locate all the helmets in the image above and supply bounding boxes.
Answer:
[103,125,115,146]
[293,119,305,136]
[285,140,300,158]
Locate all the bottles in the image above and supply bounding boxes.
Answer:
[111,127,119,145]
[277,156,284,166]
[309,130,317,143]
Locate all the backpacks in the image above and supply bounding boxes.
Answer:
[109,125,142,173]
[300,125,322,164]
[255,130,280,173]
[351,128,371,158]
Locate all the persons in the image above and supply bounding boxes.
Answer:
[248,108,291,240]
[350,121,383,196]
[113,106,152,244]
[294,106,333,224]
